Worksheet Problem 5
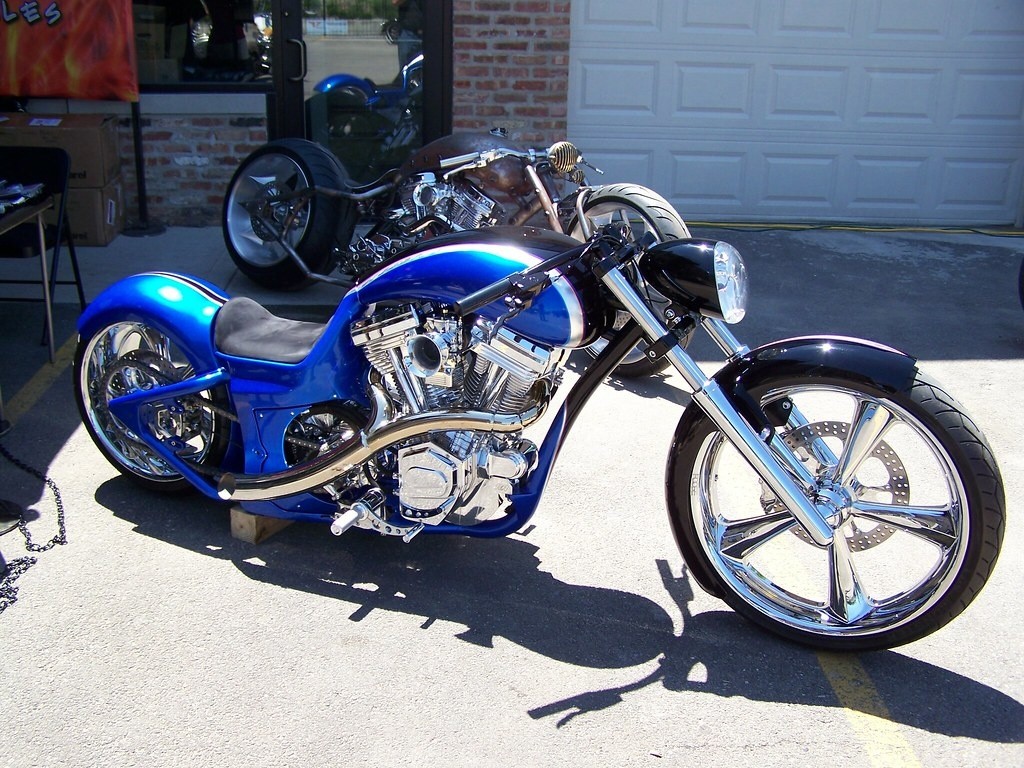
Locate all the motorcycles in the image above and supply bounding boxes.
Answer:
[308,47,426,155]
[221,135,700,380]
[72,140,1010,651]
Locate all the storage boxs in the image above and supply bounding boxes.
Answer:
[0,113,126,246]
[133,4,165,82]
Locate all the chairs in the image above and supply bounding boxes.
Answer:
[0,145,85,347]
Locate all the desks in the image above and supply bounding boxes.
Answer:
[0,203,56,363]
